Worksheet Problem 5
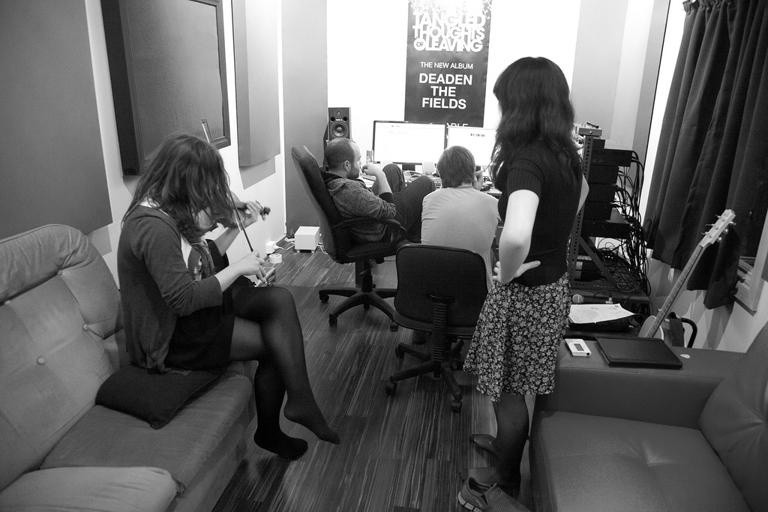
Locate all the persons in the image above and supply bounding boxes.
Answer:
[322,138,433,244]
[419,146,500,320]
[461,56,591,492]
[116,133,340,459]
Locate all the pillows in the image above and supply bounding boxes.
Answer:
[95,360,222,430]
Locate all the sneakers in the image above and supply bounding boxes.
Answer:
[456,479,530,512]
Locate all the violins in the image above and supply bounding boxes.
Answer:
[637,209,737,338]
[180,191,270,237]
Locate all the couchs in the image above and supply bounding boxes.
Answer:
[529,320,768,512]
[2,223,261,512]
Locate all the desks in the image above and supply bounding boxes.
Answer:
[568,251,650,323]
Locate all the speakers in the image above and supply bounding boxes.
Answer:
[325,107,351,144]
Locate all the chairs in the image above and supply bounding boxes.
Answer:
[383,244,490,414]
[290,143,406,330]
